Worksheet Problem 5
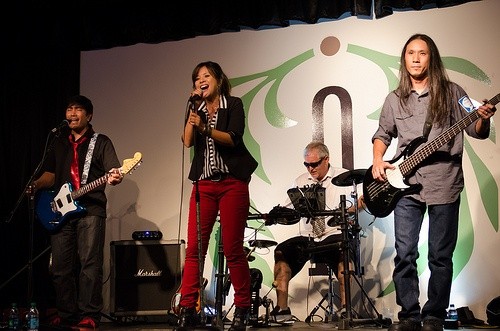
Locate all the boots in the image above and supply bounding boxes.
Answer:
[227,307,248,331]
[172,307,195,331]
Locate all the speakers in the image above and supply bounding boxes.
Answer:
[109,239,187,317]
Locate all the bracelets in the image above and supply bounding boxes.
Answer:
[200,125,212,137]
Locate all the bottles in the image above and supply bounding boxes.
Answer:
[8,303,19,331]
[447,304,458,319]
[25,302,40,331]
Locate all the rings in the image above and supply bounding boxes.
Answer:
[485,111,488,115]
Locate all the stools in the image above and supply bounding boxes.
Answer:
[305,240,343,323]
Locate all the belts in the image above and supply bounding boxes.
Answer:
[206,173,230,181]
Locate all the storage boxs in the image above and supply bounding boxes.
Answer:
[108,240,186,317]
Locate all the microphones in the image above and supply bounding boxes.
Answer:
[51,118,73,134]
[189,89,204,103]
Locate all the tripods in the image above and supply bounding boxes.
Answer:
[304,264,362,323]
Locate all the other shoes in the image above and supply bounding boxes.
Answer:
[422,320,443,331]
[340,308,353,319]
[389,317,421,331]
[262,306,292,321]
[78,317,96,328]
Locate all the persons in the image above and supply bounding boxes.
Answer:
[172,61,259,331]
[371,34,498,331]
[253,141,366,327]
[26,95,124,331]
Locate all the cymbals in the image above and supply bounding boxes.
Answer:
[331,168,369,187]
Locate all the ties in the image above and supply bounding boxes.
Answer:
[69,135,86,191]
[310,182,325,238]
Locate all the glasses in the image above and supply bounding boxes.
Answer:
[304,155,326,167]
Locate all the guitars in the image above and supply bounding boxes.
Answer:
[34,151,144,233]
[362,91,500,218]
